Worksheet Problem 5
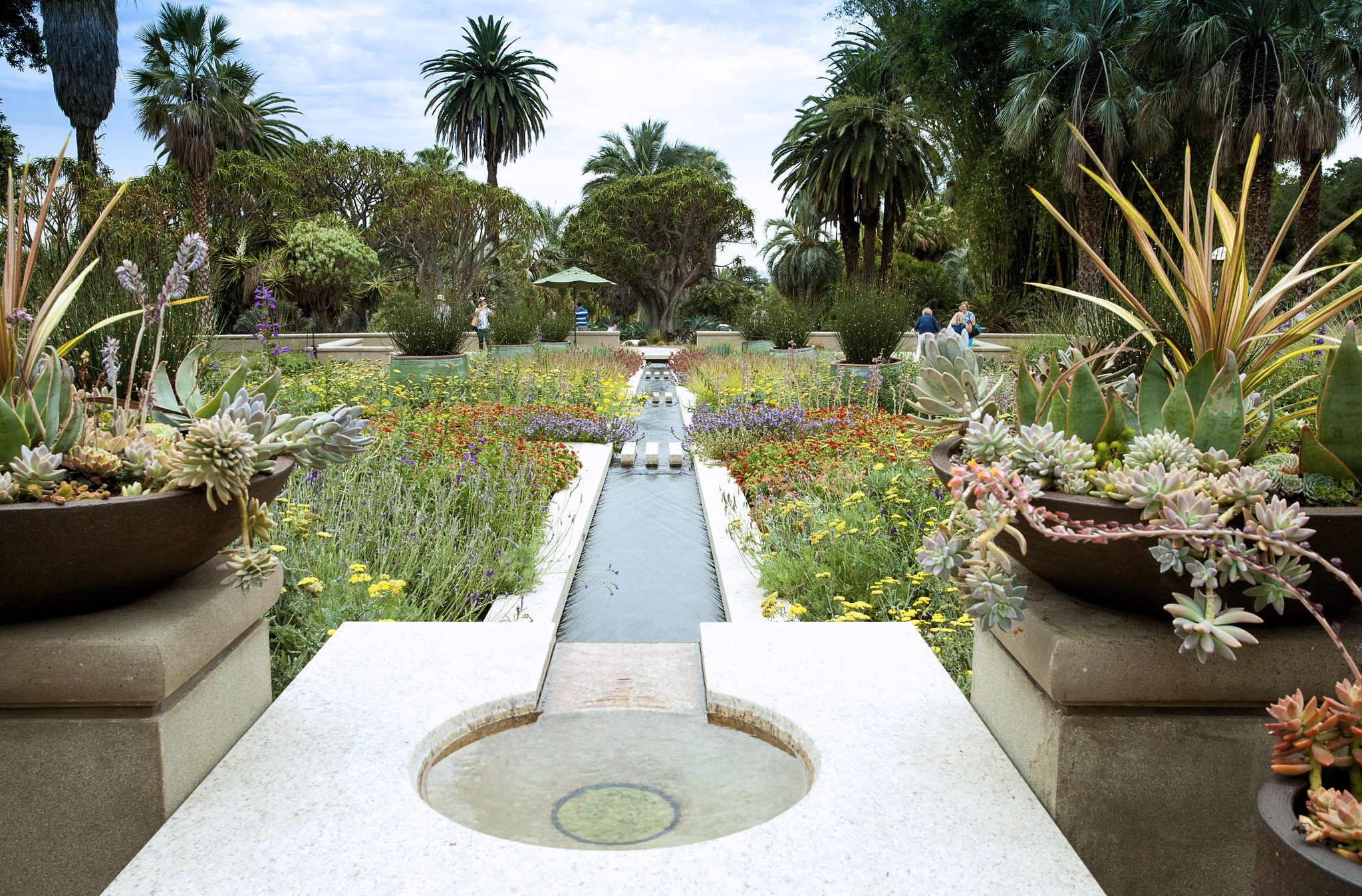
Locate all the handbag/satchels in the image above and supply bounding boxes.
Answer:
[470,311,479,327]
[967,320,980,340]
[968,311,981,336]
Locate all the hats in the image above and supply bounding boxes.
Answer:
[433,294,447,301]
[478,297,485,302]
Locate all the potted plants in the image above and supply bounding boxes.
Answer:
[827,266,916,384]
[1253,679,1362,896]
[468,295,574,359]
[371,269,479,392]
[0,125,378,622]
[916,119,1361,683]
[726,284,826,361]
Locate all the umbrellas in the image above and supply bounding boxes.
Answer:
[530,267,618,347]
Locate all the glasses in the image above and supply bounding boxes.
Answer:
[756,310,761,311]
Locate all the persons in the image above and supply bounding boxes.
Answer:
[607,320,617,331]
[547,307,556,319]
[1295,287,1313,314]
[475,297,495,352]
[959,302,976,347]
[434,294,450,327]
[1212,246,1257,309]
[575,302,588,331]
[913,307,939,363]
[750,304,767,323]
[949,311,972,336]
